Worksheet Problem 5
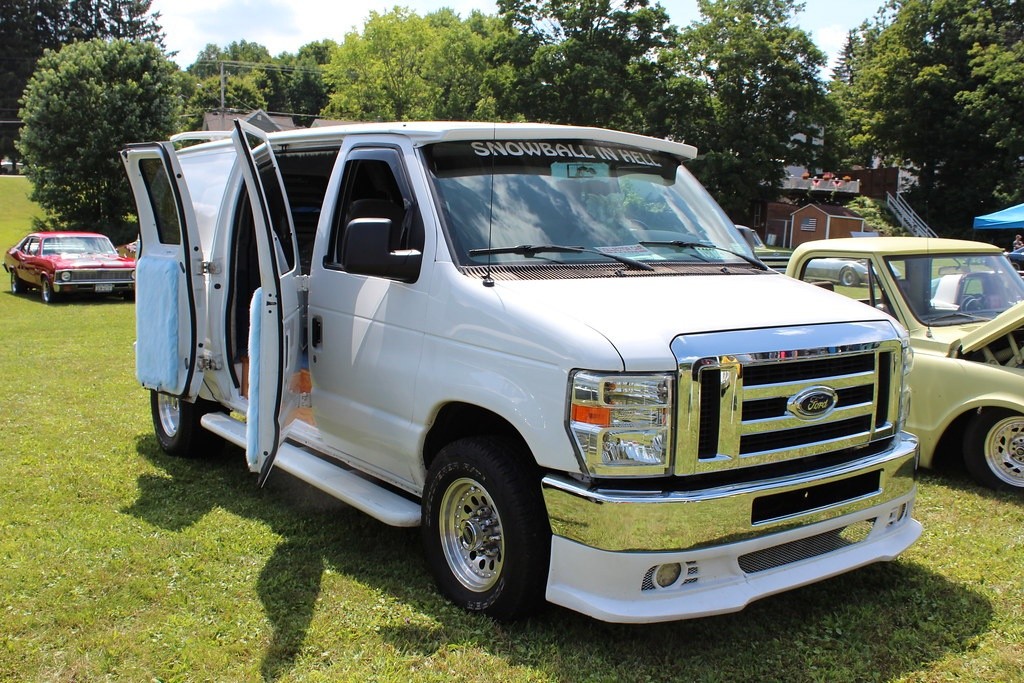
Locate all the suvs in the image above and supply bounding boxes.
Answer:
[114,115,923,625]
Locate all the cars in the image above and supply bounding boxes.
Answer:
[733,224,1024,492]
[1,230,135,304]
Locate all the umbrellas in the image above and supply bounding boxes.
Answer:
[973,203,1024,229]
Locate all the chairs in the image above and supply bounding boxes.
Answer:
[345,197,400,250]
[236,209,320,342]
[879,279,912,317]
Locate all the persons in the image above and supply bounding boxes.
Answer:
[1013,235,1024,251]
